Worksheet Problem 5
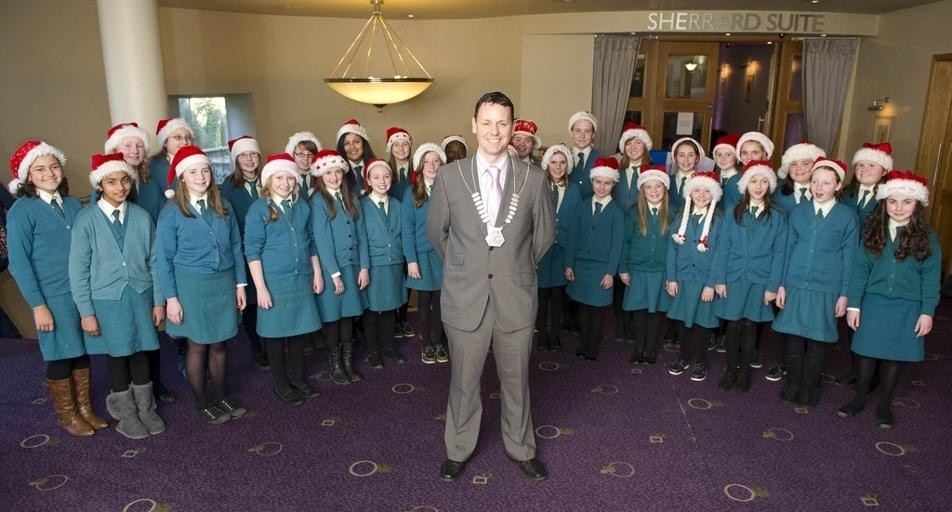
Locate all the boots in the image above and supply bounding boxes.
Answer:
[130,379,167,438]
[105,384,150,443]
[339,341,363,382]
[68,369,109,433]
[779,354,802,405]
[325,345,351,387]
[41,374,97,438]
[797,349,825,408]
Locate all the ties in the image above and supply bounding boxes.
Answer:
[484,164,504,224]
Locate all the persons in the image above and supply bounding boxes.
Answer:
[6,140,108,438]
[838,170,943,430]
[426,92,556,483]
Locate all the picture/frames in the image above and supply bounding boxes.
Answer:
[871,114,896,146]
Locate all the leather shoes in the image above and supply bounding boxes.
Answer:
[505,451,548,482]
[440,459,468,483]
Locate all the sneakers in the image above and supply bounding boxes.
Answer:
[157,386,177,404]
[288,381,322,400]
[434,342,451,366]
[384,347,406,366]
[747,348,768,368]
[837,399,867,421]
[300,331,326,357]
[194,402,230,427]
[765,363,789,383]
[214,396,249,422]
[716,364,753,394]
[689,359,711,382]
[820,369,859,394]
[875,402,894,433]
[418,341,435,366]
[667,356,690,376]
[366,349,385,371]
[250,349,270,370]
[393,320,416,339]
[533,315,729,365]
[272,382,304,408]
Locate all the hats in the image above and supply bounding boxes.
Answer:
[778,137,828,180]
[810,157,848,186]
[5,135,68,193]
[737,161,778,197]
[83,150,136,193]
[258,152,302,187]
[734,128,776,166]
[874,169,932,209]
[165,142,212,201]
[508,109,740,254]
[849,140,896,174]
[225,133,262,165]
[152,115,191,152]
[101,120,147,163]
[281,117,473,186]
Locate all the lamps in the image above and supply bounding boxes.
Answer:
[324,1,436,114]
